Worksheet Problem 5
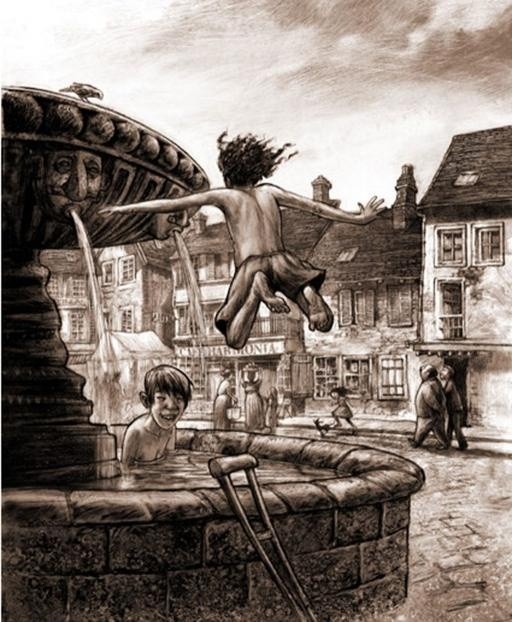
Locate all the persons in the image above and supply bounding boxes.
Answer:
[211,369,238,430]
[150,184,199,241]
[439,366,469,451]
[122,364,194,465]
[238,367,265,431]
[407,365,450,451]
[96,132,387,350]
[264,386,277,435]
[329,386,357,436]
